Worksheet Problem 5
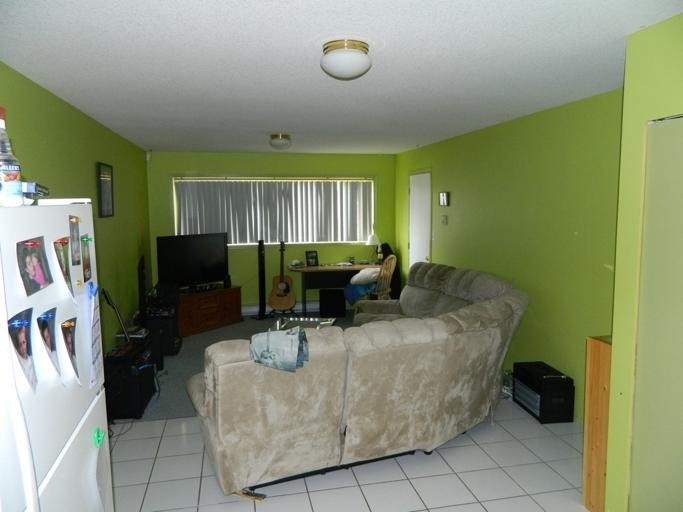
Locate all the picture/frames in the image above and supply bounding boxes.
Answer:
[95,161,114,218]
[306,251,318,266]
[438,191,448,207]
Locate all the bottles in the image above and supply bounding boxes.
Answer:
[0,118,21,197]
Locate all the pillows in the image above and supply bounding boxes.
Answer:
[349,268,390,285]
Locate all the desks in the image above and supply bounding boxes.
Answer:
[287,264,382,316]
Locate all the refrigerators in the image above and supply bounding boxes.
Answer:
[0,198,117,512]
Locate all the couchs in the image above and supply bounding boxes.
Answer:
[186,262,528,497]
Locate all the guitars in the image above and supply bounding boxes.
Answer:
[268,241,295,310]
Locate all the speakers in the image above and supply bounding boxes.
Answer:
[320,289,346,317]
[112,362,156,420]
[137,256,147,328]
[251,239,266,319]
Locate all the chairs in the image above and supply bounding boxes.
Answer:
[344,255,397,316]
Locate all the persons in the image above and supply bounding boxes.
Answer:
[15,326,37,391]
[21,248,40,296]
[61,327,78,381]
[28,252,48,289]
[39,320,58,372]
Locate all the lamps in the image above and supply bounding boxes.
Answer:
[320,39,372,79]
[365,234,383,266]
[269,135,291,148]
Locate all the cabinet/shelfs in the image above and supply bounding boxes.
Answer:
[154,284,243,337]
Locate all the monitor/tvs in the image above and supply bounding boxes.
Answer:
[156,232,228,293]
